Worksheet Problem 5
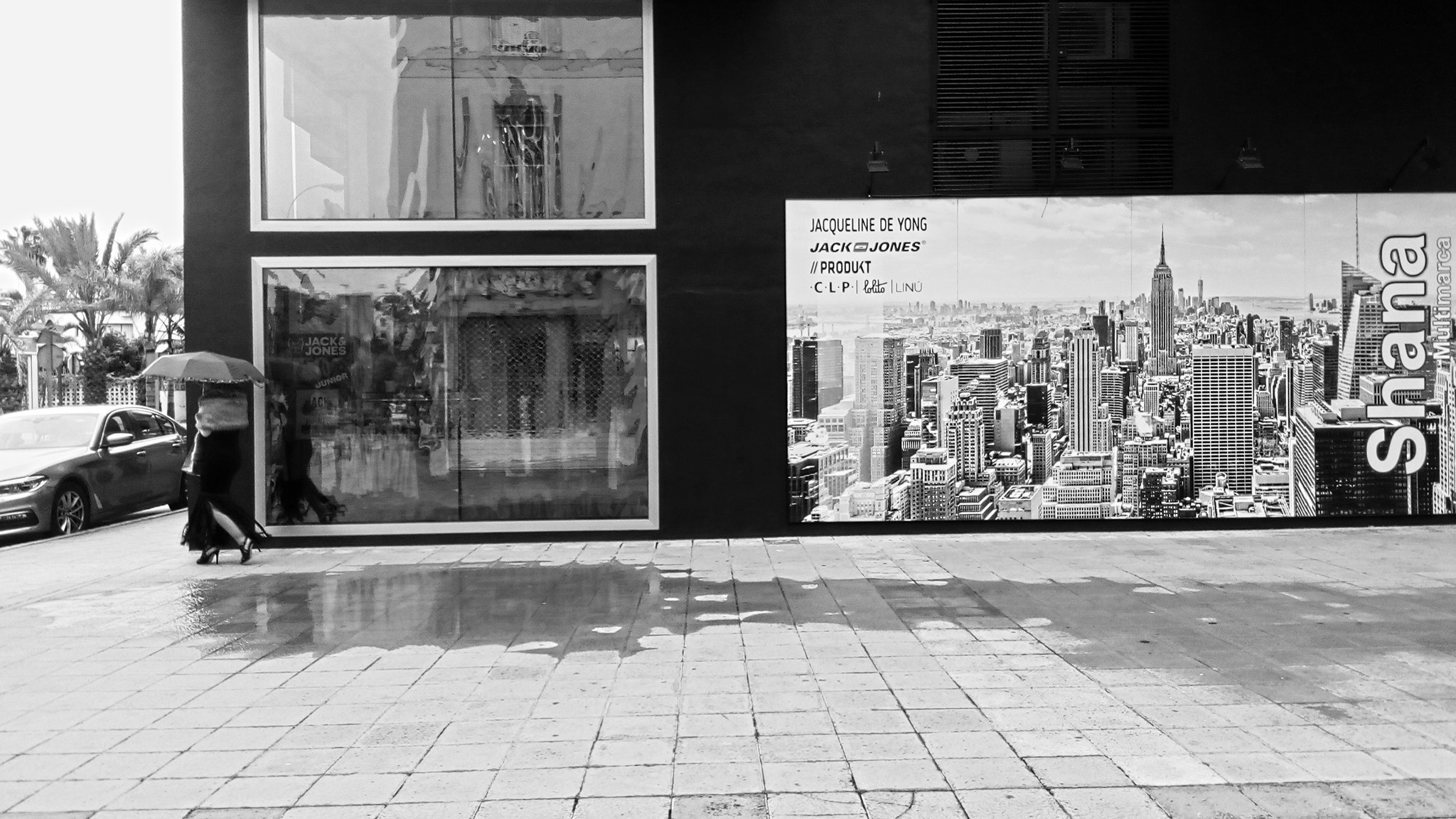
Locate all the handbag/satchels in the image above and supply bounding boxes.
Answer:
[182,431,203,478]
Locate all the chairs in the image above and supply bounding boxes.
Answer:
[136,420,151,439]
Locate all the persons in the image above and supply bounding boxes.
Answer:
[182,384,261,567]
[273,387,344,523]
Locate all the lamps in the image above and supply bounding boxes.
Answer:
[867,141,889,197]
[1218,136,1264,193]
[1385,136,1448,192]
[1051,139,1085,196]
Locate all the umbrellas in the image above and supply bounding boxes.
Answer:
[266,356,324,386]
[140,350,264,386]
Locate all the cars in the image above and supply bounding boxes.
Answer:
[0,404,190,537]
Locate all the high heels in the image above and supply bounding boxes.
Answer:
[240,536,261,564]
[196,544,221,565]
